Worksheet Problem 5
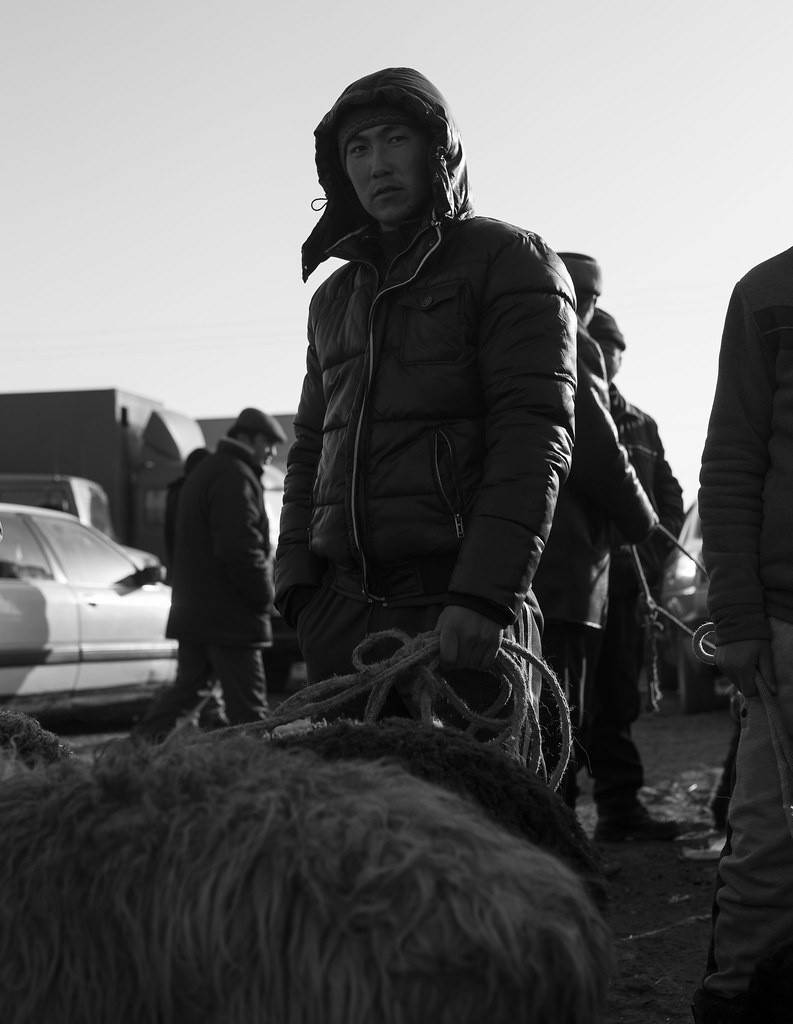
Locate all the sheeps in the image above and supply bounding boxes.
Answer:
[149,717,610,918]
[0,708,618,1023]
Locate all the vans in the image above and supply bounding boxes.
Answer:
[1,475,117,542]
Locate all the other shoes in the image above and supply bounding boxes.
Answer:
[597,817,683,845]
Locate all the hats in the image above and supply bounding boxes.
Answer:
[554,251,605,296]
[238,409,289,444]
[339,103,428,177]
[589,307,627,349]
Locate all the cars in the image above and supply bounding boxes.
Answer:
[0,506,180,739]
[645,496,733,715]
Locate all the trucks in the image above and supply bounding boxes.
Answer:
[0,387,286,610]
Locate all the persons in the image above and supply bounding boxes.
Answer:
[272,67,577,740]
[133,409,288,747]
[694,244,793,1024]
[532,252,684,841]
[585,306,685,602]
